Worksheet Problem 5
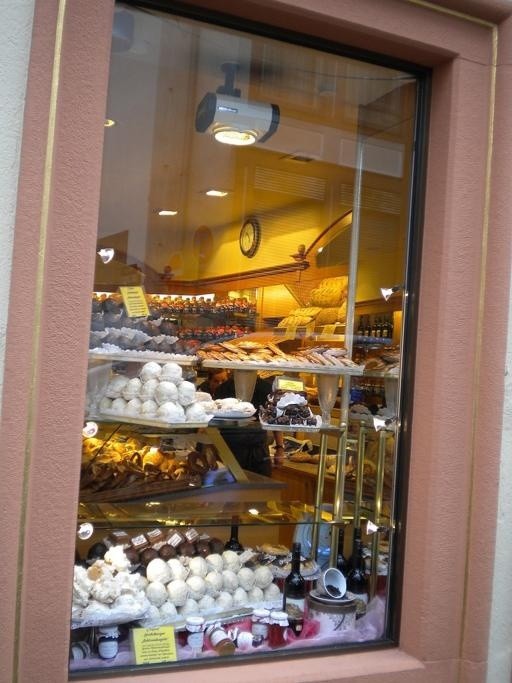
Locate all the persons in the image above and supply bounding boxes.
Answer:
[196,368,228,398]
[212,369,284,479]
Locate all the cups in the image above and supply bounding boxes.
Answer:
[316,567,346,599]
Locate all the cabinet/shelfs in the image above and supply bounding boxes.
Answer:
[78,304,286,549]
[269,293,403,545]
[156,297,269,441]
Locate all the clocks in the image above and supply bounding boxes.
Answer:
[239,217,259,259]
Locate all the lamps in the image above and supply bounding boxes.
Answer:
[79,523,94,542]
[365,520,389,539]
[373,416,399,434]
[83,419,98,438]
[96,246,114,265]
[195,59,281,149]
[380,284,404,302]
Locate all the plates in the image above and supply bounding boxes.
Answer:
[310,590,353,605]
[211,412,256,421]
[308,598,358,611]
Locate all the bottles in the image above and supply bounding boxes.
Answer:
[358,314,365,335]
[288,610,302,639]
[364,314,371,335]
[288,541,308,632]
[382,318,391,336]
[96,628,120,658]
[228,516,244,555]
[186,619,208,643]
[271,614,285,647]
[226,632,261,648]
[72,642,93,662]
[372,320,376,336]
[209,624,235,654]
[149,289,258,313]
[333,526,345,569]
[377,319,382,336]
[253,608,266,636]
[348,525,366,593]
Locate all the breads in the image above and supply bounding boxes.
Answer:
[328,349,347,356]
[243,340,262,351]
[254,354,262,364]
[278,359,289,360]
[290,306,321,315]
[241,350,249,362]
[289,355,296,365]
[338,300,349,323]
[212,350,224,359]
[300,356,312,362]
[267,341,283,355]
[311,284,340,307]
[316,308,340,324]
[197,350,209,359]
[320,275,350,290]
[312,354,322,365]
[260,351,272,362]
[344,360,357,367]
[227,351,237,360]
[278,317,310,327]
[329,356,344,366]
[218,343,238,354]
